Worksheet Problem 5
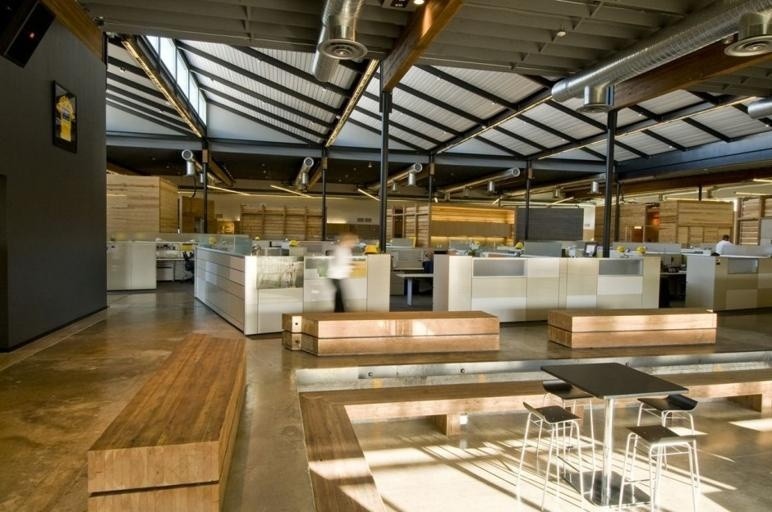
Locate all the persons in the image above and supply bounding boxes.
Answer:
[714,234,732,254]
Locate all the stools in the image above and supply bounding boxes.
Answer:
[515,363,701,512]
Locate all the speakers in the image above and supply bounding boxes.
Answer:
[0,0,56,68]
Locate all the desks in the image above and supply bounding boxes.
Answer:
[157,257,195,288]
[395,272,433,306]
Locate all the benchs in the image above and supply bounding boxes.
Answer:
[87,331,247,512]
[303,370,772,438]
[548,306,718,349]
[300,393,383,512]
[282,311,499,357]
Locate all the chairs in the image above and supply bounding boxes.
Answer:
[181,252,195,283]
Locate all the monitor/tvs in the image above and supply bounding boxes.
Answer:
[583,242,599,257]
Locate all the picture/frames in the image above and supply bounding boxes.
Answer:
[52,81,79,155]
